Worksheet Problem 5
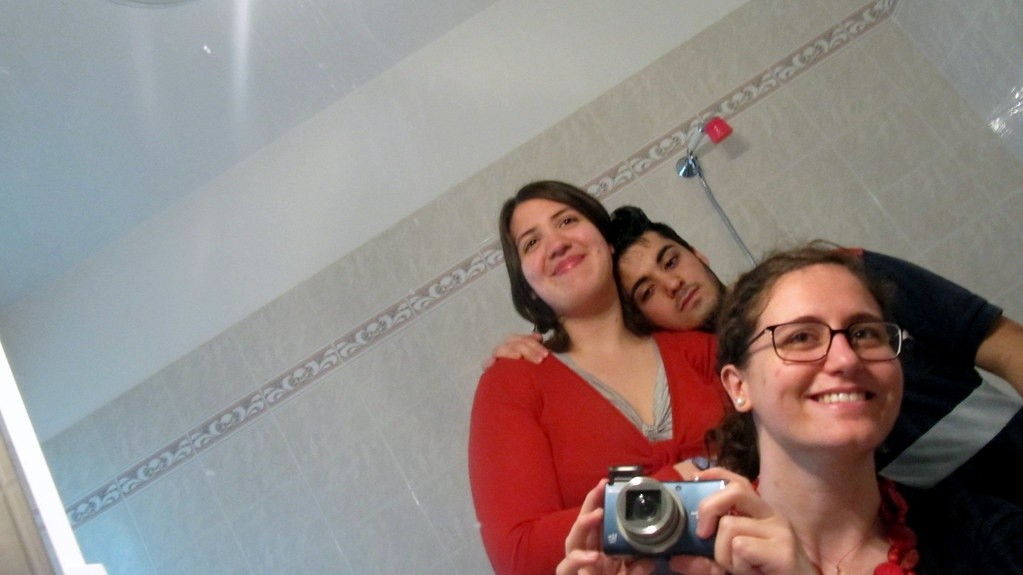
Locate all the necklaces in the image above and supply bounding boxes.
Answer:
[810,519,877,575]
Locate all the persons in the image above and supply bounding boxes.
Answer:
[468,181,736,575]
[481,206,1023,575]
[555,248,919,575]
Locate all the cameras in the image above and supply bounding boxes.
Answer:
[602,475,729,557]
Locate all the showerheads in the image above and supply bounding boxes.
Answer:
[686,114,733,159]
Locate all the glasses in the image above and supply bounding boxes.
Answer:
[730,320,912,363]
